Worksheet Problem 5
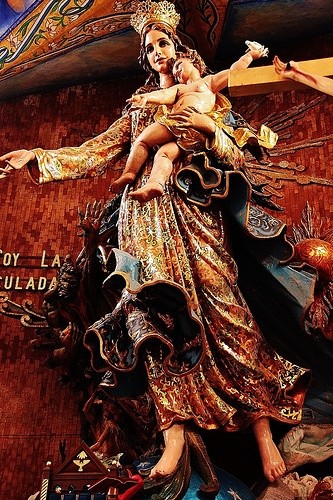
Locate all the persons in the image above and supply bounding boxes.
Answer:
[0,1,311,483]
[39,199,108,363]
[82,370,128,456]
[273,55,333,96]
[109,45,270,204]
